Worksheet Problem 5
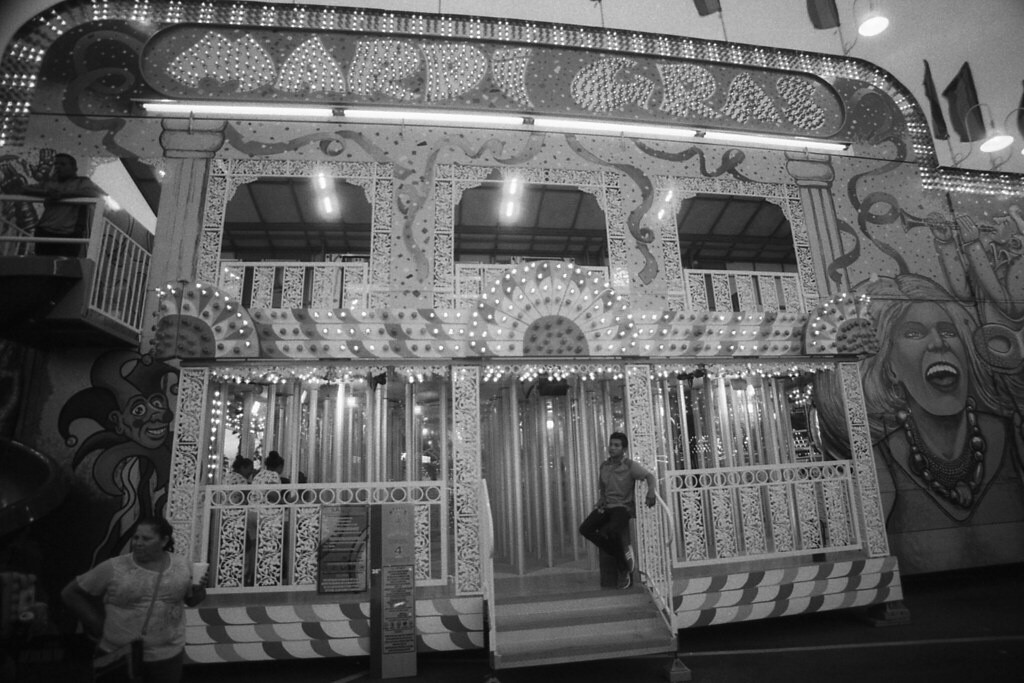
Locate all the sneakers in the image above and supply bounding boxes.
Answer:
[624,544,634,573]
[617,571,631,590]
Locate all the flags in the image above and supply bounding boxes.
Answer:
[942,61,987,142]
[807,0,840,29]
[922,59,949,140]
[694,0,721,16]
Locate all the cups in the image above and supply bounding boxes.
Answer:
[192,562,209,585]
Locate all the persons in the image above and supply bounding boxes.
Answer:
[250,450,284,509]
[580,431,656,590]
[23,154,107,255]
[61,517,206,683]
[223,456,254,505]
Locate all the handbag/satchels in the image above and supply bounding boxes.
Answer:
[91,637,143,683]
